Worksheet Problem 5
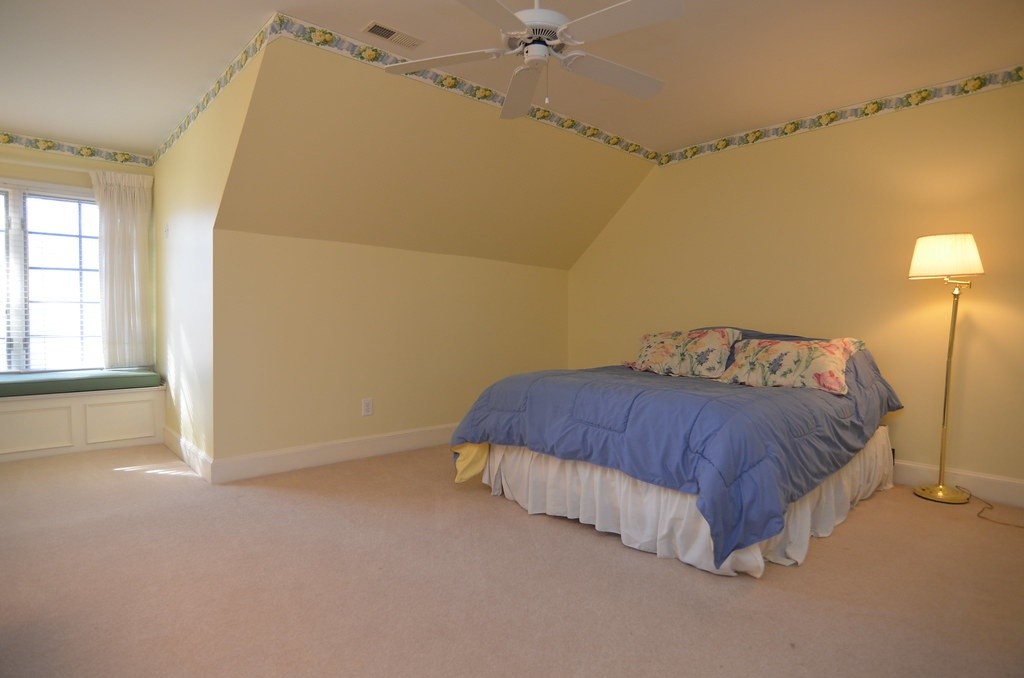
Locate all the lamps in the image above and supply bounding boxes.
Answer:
[908,233,985,504]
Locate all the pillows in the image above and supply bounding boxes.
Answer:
[710,336,866,395]
[622,328,742,378]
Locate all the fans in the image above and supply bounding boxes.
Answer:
[383,0,682,118]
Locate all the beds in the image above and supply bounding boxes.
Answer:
[451,327,905,581]
